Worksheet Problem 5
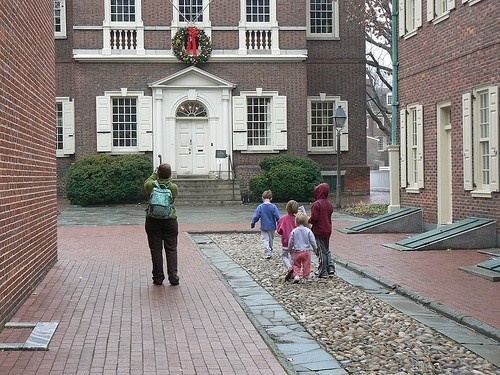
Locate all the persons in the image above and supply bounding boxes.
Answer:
[277,200,299,280]
[308,183,336,278]
[286,213,318,284]
[296,206,312,228]
[144,163,179,285]
[250,190,280,259]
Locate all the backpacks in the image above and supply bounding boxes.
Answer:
[147,180,174,219]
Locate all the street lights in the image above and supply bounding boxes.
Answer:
[331,105,347,209]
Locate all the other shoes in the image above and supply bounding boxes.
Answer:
[266,255,271,259]
[153,280,161,285]
[319,272,327,278]
[329,267,335,274]
[171,282,178,285]
[284,268,293,280]
[302,278,307,284]
[293,275,300,283]
